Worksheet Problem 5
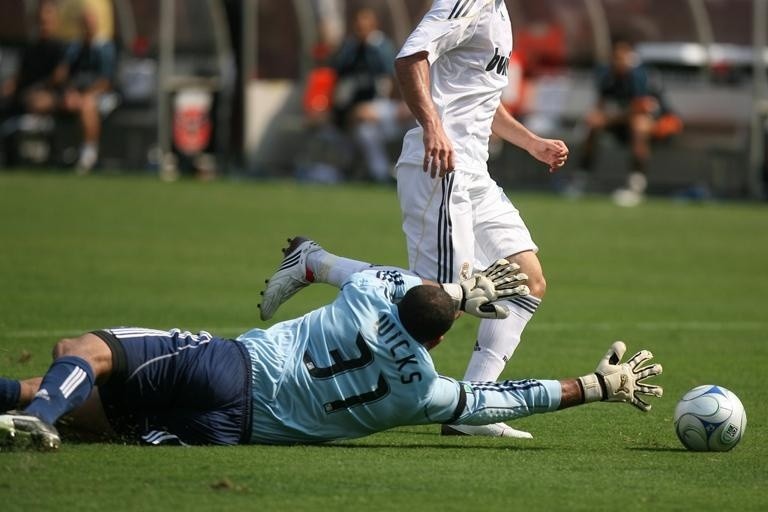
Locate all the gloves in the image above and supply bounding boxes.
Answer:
[578,339,664,413]
[440,258,528,320]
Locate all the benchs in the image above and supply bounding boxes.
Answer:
[102,92,158,165]
[661,115,753,200]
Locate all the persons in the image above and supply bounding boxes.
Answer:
[25,1,120,175]
[558,37,682,207]
[1,255,666,451]
[2,3,68,168]
[321,6,413,183]
[257,0,570,440]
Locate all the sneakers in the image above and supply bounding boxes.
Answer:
[1,414,79,453]
[441,409,533,442]
[258,236,321,321]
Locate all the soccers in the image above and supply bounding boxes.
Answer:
[676,386,745,453]
